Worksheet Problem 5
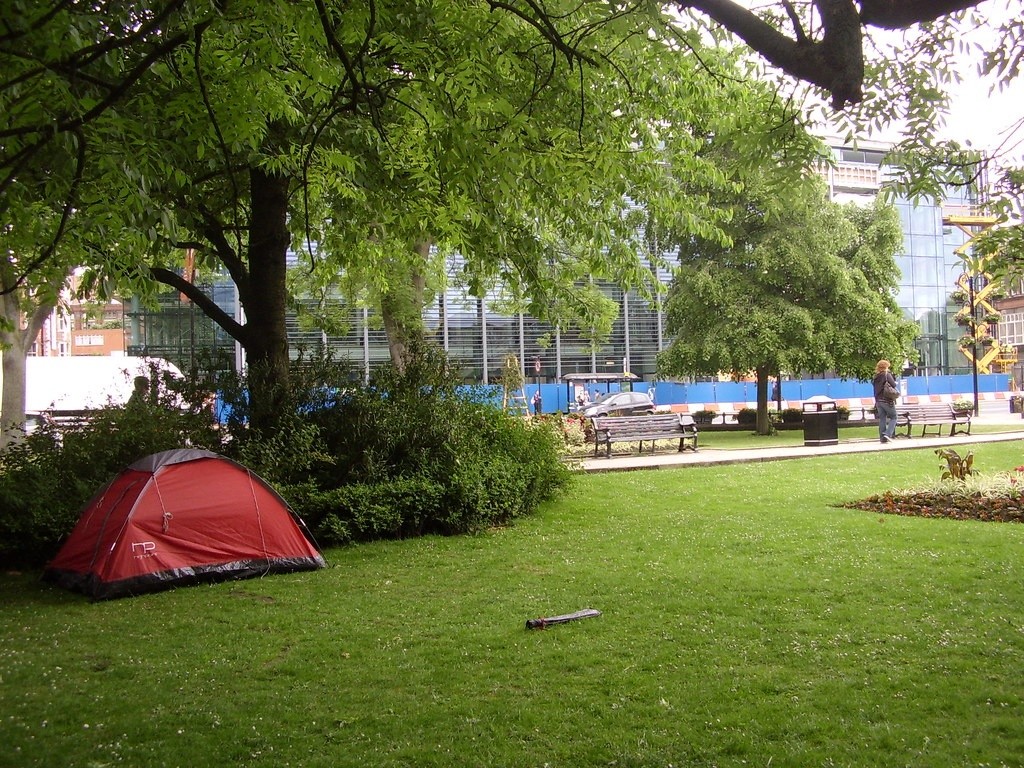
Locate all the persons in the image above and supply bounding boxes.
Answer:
[871,360,899,443]
[533,391,540,415]
[127,376,150,405]
[577,389,600,407]
[771,381,779,401]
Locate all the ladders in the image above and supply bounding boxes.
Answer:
[502,357,530,417]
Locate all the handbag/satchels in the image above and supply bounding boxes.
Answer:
[883,373,900,399]
[531,397,535,404]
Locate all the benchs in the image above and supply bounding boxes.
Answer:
[891,403,973,439]
[591,412,699,459]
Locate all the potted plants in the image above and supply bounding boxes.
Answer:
[738,409,757,423]
[954,399,974,417]
[693,410,717,425]
[836,405,850,420]
[783,408,803,422]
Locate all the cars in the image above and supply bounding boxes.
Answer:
[579,392,656,418]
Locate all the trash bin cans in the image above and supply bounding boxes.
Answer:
[802,401,838,446]
[1010,396,1022,413]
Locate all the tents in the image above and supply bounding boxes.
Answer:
[39,448,326,602]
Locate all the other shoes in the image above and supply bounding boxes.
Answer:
[884,435,893,443]
[882,441,891,444]
